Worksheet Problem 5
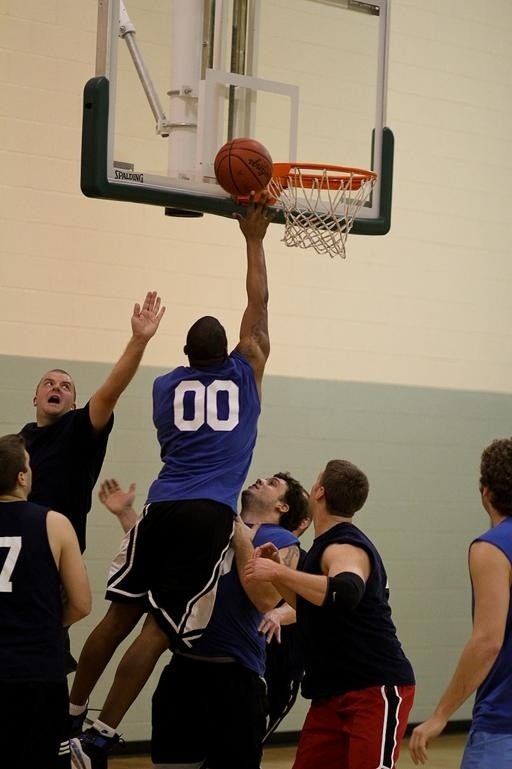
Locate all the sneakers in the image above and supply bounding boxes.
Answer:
[69,707,120,769]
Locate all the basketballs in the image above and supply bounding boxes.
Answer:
[215,138,273,196]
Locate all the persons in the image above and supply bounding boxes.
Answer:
[0,433,93,769]
[250,459,416,769]
[18,290,166,675]
[67,190,281,769]
[407,434,512,769]
[98,471,306,769]
[262,489,312,742]
